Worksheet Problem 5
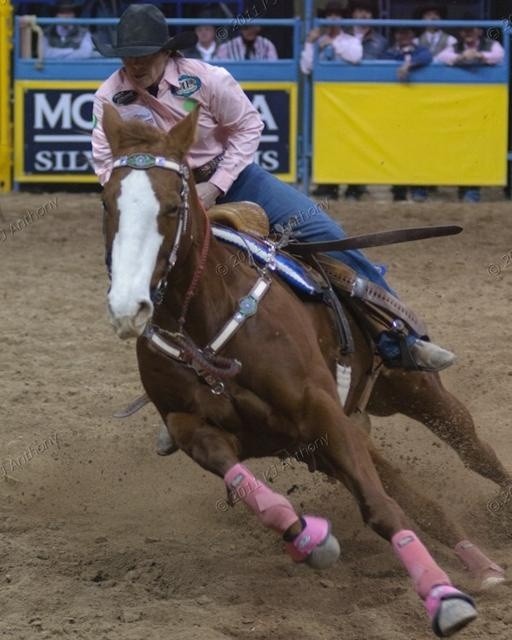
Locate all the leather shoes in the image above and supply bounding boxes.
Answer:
[382,339,456,373]
[155,427,177,456]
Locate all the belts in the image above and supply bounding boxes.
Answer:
[192,156,224,184]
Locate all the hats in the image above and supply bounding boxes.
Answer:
[91,3,198,58]
[410,2,448,34]
[316,1,349,18]
[347,0,379,20]
[45,0,82,17]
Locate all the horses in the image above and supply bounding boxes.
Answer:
[100,101,512,639]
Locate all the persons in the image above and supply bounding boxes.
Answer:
[436,9,511,202]
[299,3,456,202]
[88,4,457,456]
[35,1,129,59]
[186,8,278,61]
